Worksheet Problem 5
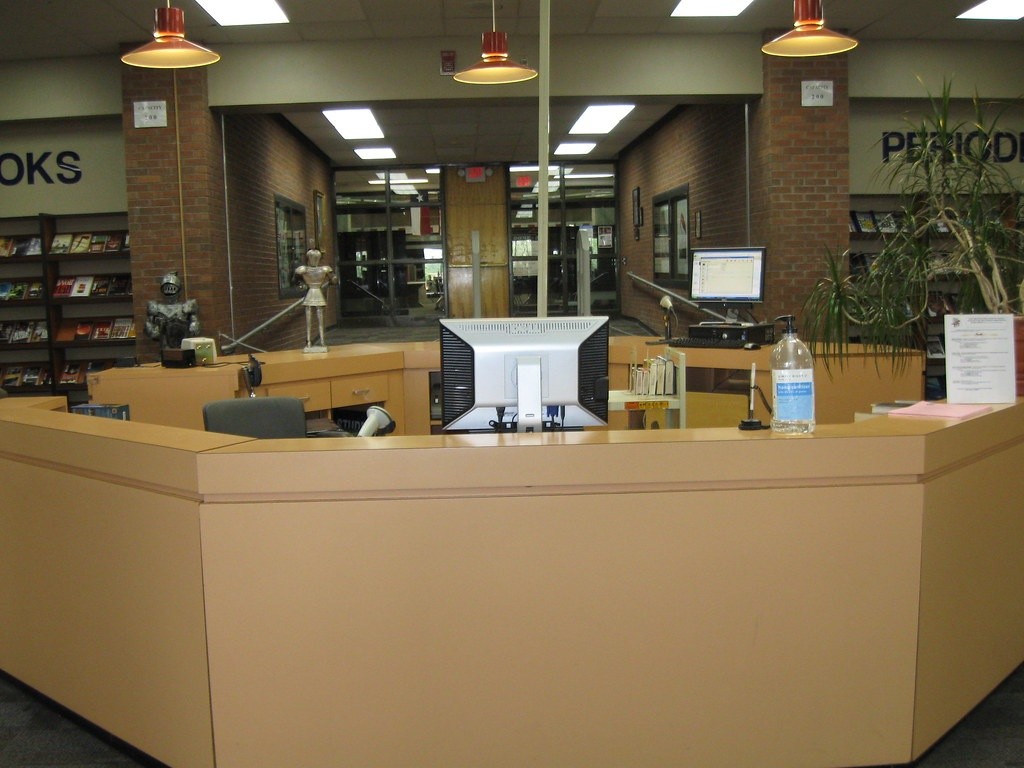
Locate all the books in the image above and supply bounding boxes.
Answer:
[870,400,918,413]
[632,355,674,395]
[849,211,975,400]
[306,419,343,437]
[0,232,136,385]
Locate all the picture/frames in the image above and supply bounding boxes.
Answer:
[632,187,640,226]
[639,207,642,227]
[313,189,326,258]
[694,210,701,238]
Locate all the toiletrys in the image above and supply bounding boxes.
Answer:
[768,313,818,436]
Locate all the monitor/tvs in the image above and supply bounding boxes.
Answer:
[688,246,768,327]
[439,315,610,433]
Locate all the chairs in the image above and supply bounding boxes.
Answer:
[202,395,307,440]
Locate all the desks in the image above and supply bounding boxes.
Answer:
[426,292,444,309]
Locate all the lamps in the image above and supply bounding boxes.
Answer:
[453,0,537,85]
[458,167,493,182]
[761,0,857,57]
[121,0,220,69]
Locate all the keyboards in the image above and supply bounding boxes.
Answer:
[669,337,746,349]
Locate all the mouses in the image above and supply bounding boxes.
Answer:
[744,342,760,350]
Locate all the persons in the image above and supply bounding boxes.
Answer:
[926,296,950,316]
[292,249,338,347]
[142,271,200,351]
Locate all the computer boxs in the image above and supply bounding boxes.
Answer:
[688,324,776,345]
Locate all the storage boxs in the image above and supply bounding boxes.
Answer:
[71,404,130,421]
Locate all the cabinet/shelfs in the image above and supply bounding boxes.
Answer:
[608,347,686,429]
[849,210,1024,391]
[268,375,389,421]
[0,211,136,413]
